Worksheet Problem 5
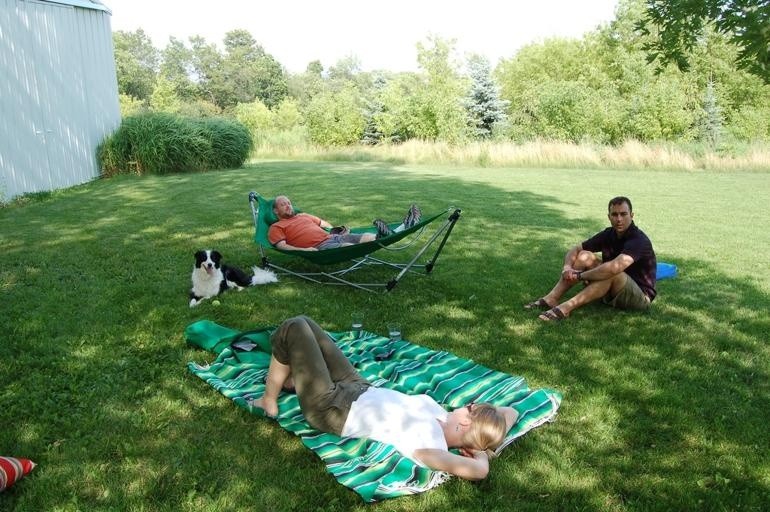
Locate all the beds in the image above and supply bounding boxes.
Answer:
[249,189,461,297]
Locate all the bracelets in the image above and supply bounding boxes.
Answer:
[575,270,582,281]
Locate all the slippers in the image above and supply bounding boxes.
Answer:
[246,397,278,418]
[263,374,296,394]
[538,306,568,322]
[523,297,553,310]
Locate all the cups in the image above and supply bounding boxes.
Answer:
[387,322,402,340]
[351,312,365,328]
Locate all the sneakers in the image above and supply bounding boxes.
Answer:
[402,204,421,230]
[372,218,395,237]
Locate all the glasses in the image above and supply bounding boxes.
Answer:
[465,401,475,413]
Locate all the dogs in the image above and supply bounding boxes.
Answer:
[188,250,279,309]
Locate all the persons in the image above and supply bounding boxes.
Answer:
[522,195,657,322]
[247,313,519,482]
[267,193,424,252]
